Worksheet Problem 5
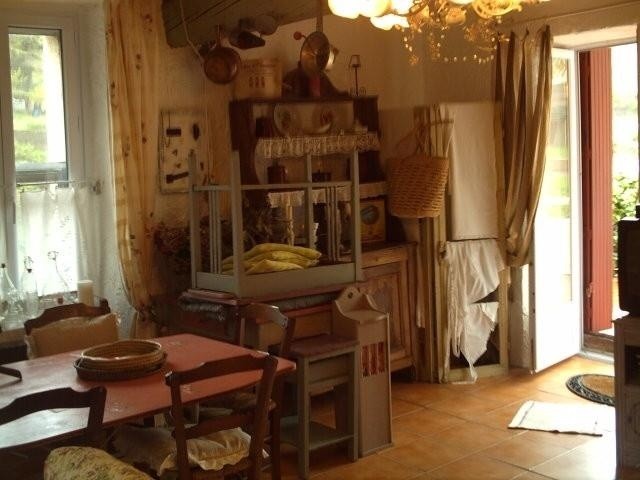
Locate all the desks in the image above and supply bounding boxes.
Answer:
[184,284,351,416]
[605,314,639,480]
[184,148,366,296]
[1,330,297,453]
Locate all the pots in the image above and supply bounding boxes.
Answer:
[204,25,241,85]
[292,30,331,73]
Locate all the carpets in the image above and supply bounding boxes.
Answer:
[564,371,617,408]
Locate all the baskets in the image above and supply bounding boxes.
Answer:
[385,119,450,219]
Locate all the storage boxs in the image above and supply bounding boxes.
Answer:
[335,195,389,247]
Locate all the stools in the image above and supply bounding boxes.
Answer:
[268,332,361,479]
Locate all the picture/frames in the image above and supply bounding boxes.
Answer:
[159,105,215,195]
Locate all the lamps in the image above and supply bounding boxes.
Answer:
[326,0,524,69]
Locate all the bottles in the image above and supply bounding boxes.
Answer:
[0,249,68,327]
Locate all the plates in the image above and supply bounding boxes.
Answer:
[274,103,303,137]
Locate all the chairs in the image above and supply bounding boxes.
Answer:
[0,384,107,473]
[22,297,118,361]
[108,352,279,478]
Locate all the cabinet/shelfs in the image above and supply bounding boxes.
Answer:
[220,235,427,407]
[227,96,392,253]
